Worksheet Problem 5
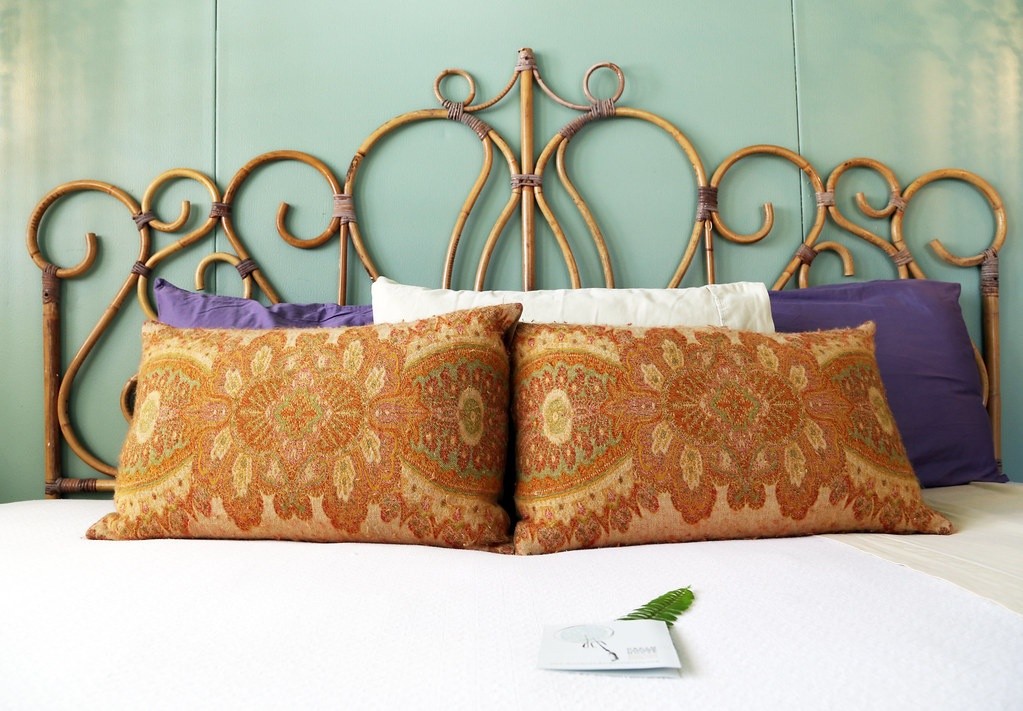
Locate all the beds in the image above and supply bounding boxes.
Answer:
[2,45,1023,554]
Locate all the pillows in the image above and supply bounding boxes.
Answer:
[364,277,778,337]
[151,277,372,329]
[87,301,524,553]
[760,277,1012,488]
[507,319,957,552]
